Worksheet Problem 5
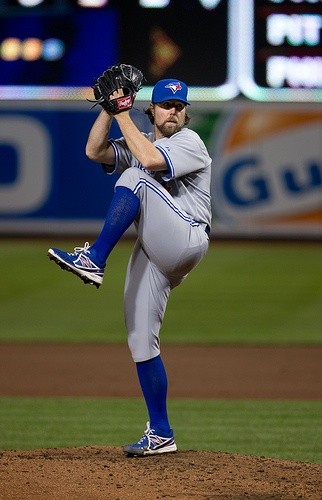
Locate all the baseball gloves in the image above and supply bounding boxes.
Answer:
[83,63,146,115]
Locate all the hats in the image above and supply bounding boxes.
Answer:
[152,78,193,107]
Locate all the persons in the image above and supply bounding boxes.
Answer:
[46,65,212,460]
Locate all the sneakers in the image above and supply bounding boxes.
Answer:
[123,427,179,457]
[46,248,105,290]
